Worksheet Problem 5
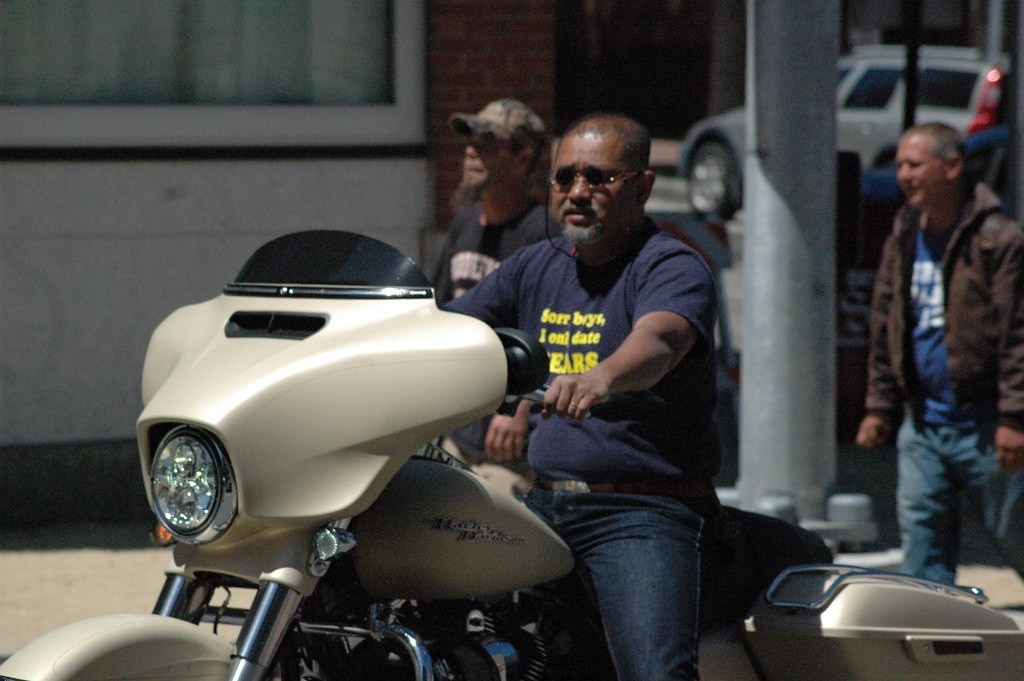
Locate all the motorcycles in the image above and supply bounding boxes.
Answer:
[0,226,1024,680]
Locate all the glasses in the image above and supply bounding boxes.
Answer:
[550,164,645,187]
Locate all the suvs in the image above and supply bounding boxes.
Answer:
[675,40,1013,217]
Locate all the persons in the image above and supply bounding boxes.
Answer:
[436,113,719,681]
[432,89,562,469]
[856,122,1024,589]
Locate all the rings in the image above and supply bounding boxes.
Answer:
[571,402,577,408]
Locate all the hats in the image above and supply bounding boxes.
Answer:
[451,98,546,149]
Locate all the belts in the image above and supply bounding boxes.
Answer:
[540,476,709,498]
